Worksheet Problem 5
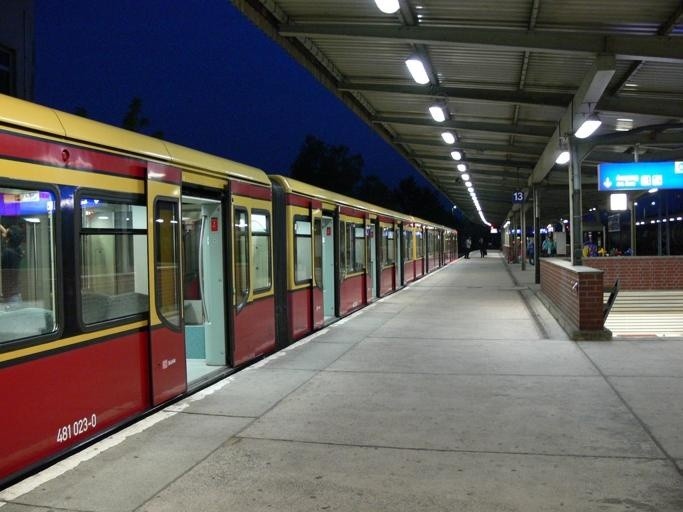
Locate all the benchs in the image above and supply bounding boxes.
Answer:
[0,290,149,343]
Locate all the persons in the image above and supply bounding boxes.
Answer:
[546,236,555,257]
[540,236,548,256]
[513,238,520,263]
[477,237,486,259]
[586,236,598,257]
[0,220,28,306]
[462,236,472,259]
[526,238,533,265]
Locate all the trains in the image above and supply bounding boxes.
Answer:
[0,91,461,489]
